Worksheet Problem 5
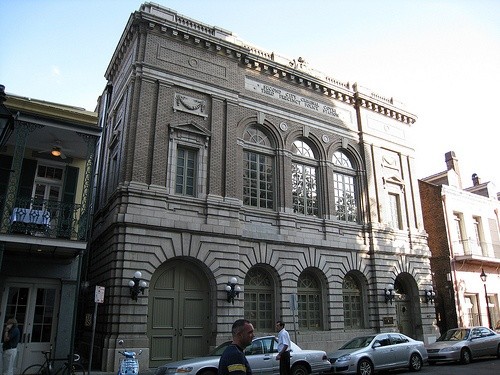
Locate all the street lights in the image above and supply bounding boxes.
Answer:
[479,268,494,331]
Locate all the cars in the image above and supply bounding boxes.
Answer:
[156,334,331,375]
[324,332,428,375]
[424,326,500,363]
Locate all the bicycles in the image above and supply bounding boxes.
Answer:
[21,349,85,375]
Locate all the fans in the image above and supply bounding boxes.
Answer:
[37,141,74,159]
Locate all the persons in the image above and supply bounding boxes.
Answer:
[2,319,20,375]
[275,321,292,375]
[219,319,255,375]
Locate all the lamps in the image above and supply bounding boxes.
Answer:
[384,284,396,305]
[425,289,435,305]
[225,277,241,304]
[128,271,149,302]
[51,150,62,157]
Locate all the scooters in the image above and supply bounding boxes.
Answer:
[117,339,143,375]
